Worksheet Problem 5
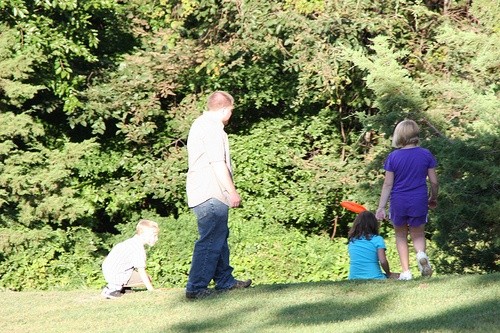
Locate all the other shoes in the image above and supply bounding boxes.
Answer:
[103,289,122,299]
[131,284,147,290]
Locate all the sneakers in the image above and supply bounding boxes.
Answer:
[398,271,412,280]
[215,279,252,290]
[186,289,222,297]
[417,252,432,276]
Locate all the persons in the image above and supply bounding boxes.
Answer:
[101,219,160,299]
[348,211,400,280]
[186,91,252,299]
[375,119,439,281]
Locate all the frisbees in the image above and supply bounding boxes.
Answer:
[340,200,367,213]
